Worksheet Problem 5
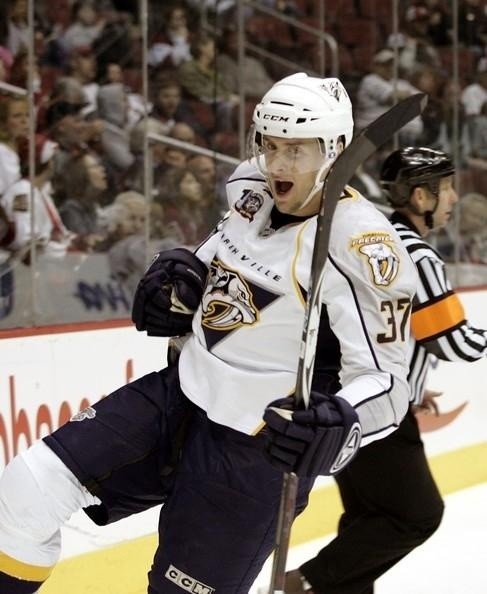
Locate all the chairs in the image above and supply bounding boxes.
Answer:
[0,0,487,247]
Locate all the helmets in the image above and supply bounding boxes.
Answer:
[379,146,457,208]
[250,71,355,149]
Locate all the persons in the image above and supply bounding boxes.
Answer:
[1,0,486,262]
[1,68,417,594]
[283,146,486,594]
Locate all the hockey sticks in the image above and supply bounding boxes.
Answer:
[268,92,429,594]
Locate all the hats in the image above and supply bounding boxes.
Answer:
[371,50,397,65]
[17,133,59,168]
[45,100,90,126]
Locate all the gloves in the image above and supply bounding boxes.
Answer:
[131,247,211,338]
[261,390,364,477]
